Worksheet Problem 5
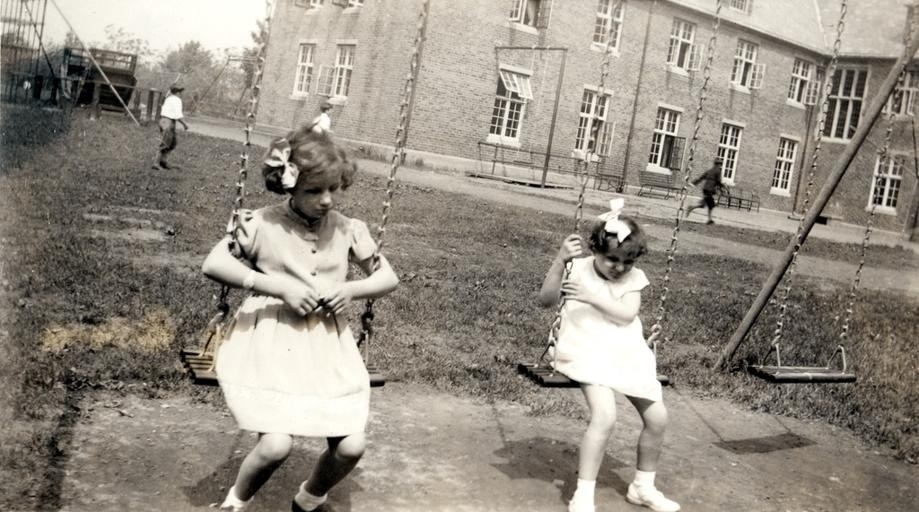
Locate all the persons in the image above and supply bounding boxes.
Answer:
[152,84,188,171]
[203,125,399,512]
[538,199,682,512]
[310,103,333,135]
[684,156,726,225]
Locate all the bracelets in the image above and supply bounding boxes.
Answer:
[243,269,256,292]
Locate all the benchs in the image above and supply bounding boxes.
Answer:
[594,160,627,192]
[721,183,761,213]
[637,170,683,199]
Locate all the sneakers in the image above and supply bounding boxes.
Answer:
[152,160,171,170]
[685,205,692,217]
[568,491,595,512]
[291,498,332,511]
[626,483,681,512]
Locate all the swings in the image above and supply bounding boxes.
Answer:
[179,0,431,386]
[747,2,918,384]
[518,2,721,389]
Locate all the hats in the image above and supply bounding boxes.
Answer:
[170,83,184,91]
[714,157,721,164]
